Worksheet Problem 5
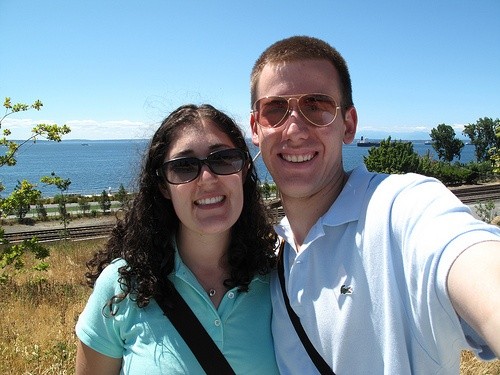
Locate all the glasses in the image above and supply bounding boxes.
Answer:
[156,148,245,185]
[250,93,354,129]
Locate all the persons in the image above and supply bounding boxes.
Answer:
[250,35,500,375]
[75,104,279,374]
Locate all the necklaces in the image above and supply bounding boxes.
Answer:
[208,288,216,297]
